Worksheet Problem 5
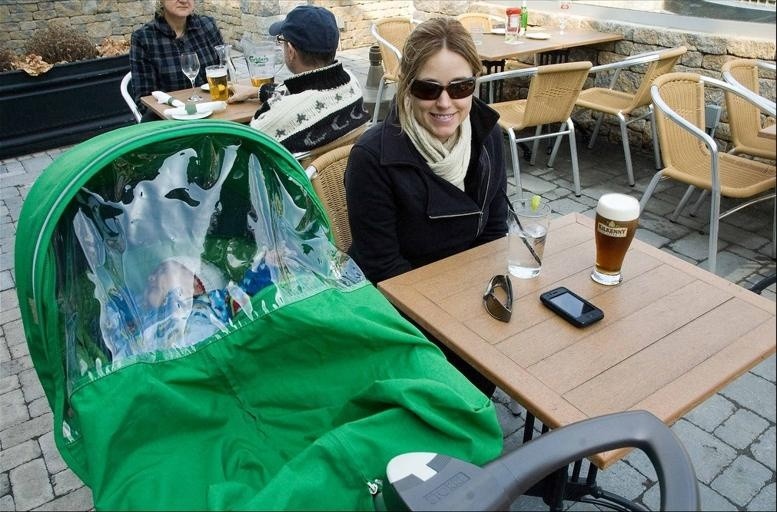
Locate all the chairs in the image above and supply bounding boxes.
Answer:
[305,145,354,253]
[119,70,143,124]
[293,120,370,170]
[372,15,414,126]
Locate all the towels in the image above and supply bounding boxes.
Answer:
[152,91,185,107]
[164,100,227,116]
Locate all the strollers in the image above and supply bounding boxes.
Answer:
[15,114,704,510]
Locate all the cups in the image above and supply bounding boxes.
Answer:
[470,0,569,45]
[589,193,642,286]
[507,198,551,281]
[171,41,284,102]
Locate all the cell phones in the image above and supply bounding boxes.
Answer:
[540,287,604,328]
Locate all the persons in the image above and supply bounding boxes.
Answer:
[226,5,373,154]
[130,0,236,124]
[343,17,509,399]
[95,240,297,363]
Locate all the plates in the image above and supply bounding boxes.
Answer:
[170,104,214,120]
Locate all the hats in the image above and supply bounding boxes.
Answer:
[154,253,229,295]
[266,5,340,56]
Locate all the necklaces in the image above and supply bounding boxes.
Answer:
[175,28,185,38]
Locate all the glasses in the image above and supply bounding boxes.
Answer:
[276,35,298,51]
[410,74,476,100]
